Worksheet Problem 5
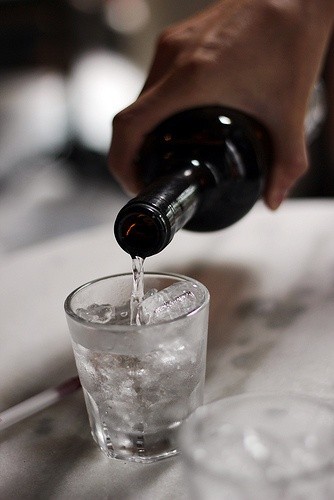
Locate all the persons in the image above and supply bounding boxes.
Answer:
[106,0,334,211]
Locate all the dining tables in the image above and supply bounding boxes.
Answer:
[1,160,334,499]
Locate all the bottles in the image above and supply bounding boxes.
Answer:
[112,105,274,257]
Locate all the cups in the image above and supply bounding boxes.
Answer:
[177,394,332,500]
[65,272,211,466]
